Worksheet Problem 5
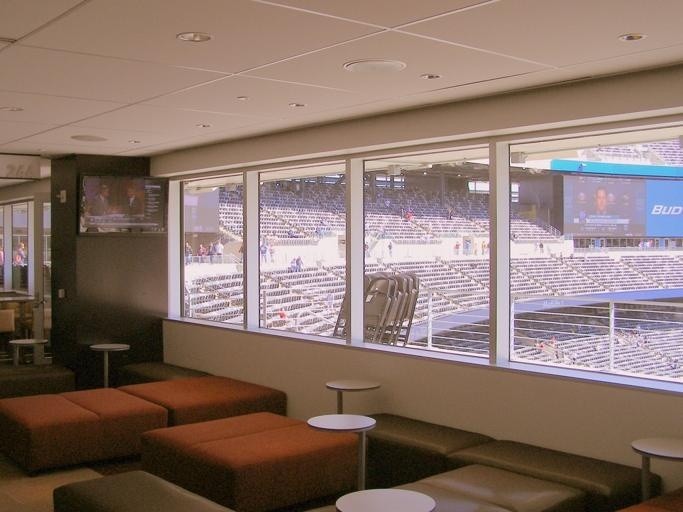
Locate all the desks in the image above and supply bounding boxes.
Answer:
[88,342,130,389]
[0,294,35,353]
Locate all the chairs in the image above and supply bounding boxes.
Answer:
[0,309,16,352]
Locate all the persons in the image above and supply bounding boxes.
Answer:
[12,243,26,268]
[127,187,138,209]
[91,184,110,216]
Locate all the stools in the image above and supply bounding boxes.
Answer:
[7,338,48,365]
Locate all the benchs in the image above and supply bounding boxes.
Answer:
[1,363,681,511]
